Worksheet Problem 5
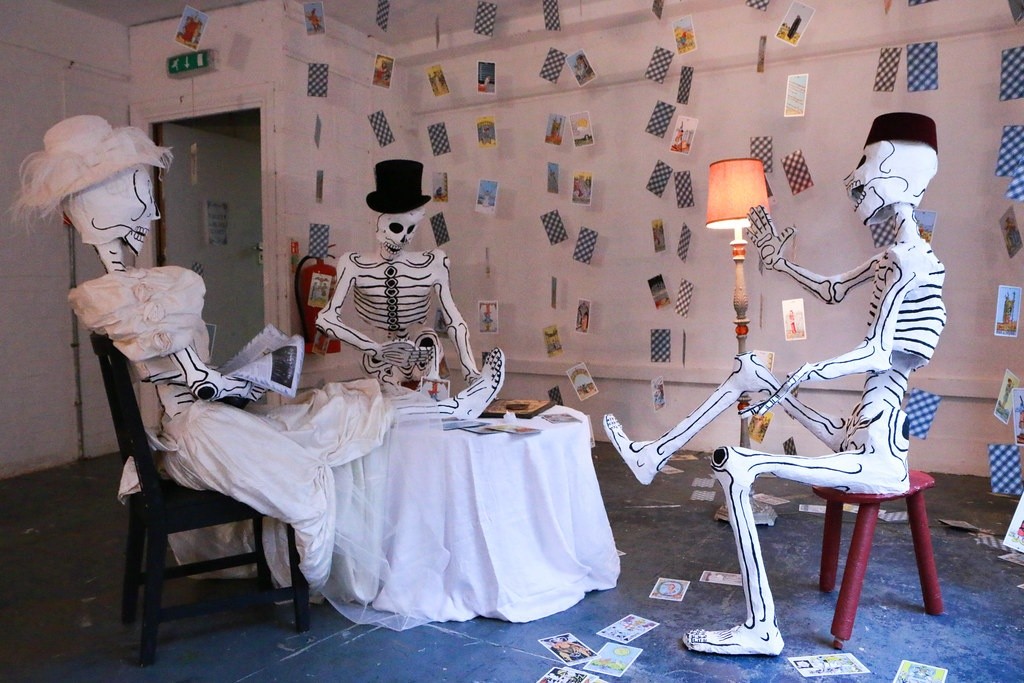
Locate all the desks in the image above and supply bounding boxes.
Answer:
[251,398,621,631]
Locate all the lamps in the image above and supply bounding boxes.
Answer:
[706,159,778,526]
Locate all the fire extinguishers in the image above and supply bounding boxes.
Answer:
[294,243,345,359]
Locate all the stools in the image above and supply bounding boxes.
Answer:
[812,468,944,651]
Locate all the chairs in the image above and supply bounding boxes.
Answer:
[90,331,309,665]
